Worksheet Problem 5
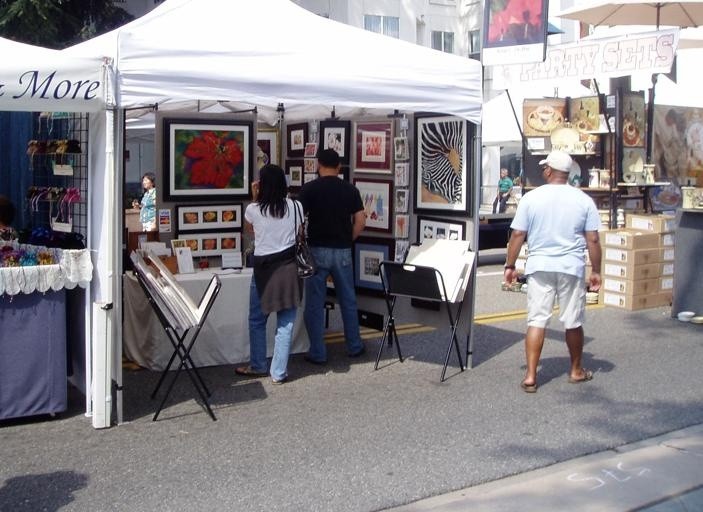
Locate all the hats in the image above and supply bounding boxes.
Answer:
[538,149,572,172]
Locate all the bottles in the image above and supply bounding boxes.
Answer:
[680,188,692,209]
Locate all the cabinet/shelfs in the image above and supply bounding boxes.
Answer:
[520,87,655,230]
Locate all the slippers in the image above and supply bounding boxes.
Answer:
[568,368,591,383]
[520,378,537,393]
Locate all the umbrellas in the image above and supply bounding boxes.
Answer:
[557,0,702,166]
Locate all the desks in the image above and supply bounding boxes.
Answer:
[123,265,311,373]
[0,240,95,424]
[672,207,703,322]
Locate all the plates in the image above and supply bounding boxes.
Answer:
[527,110,564,132]
[623,173,636,182]
[622,124,641,147]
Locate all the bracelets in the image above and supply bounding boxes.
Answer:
[505,264,516,269]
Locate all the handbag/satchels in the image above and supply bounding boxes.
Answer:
[292,199,318,279]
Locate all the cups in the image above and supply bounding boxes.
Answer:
[585,141,593,152]
[133,200,138,209]
[538,105,554,126]
[642,165,655,183]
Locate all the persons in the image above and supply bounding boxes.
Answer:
[503,151,601,395]
[130,171,158,233]
[233,164,304,383]
[493,167,513,214]
[298,149,374,365]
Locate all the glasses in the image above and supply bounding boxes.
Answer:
[542,166,548,171]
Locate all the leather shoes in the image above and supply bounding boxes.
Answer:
[271,379,283,385]
[348,343,365,357]
[304,355,328,366]
[235,367,268,377]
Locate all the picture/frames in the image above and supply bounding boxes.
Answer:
[171,239,189,254]
[351,119,395,176]
[255,127,282,183]
[395,136,410,263]
[412,111,473,216]
[161,117,255,201]
[174,203,243,257]
[417,216,467,250]
[353,239,392,292]
[352,178,395,233]
[285,122,351,195]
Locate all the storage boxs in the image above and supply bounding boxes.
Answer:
[508,201,674,312]
